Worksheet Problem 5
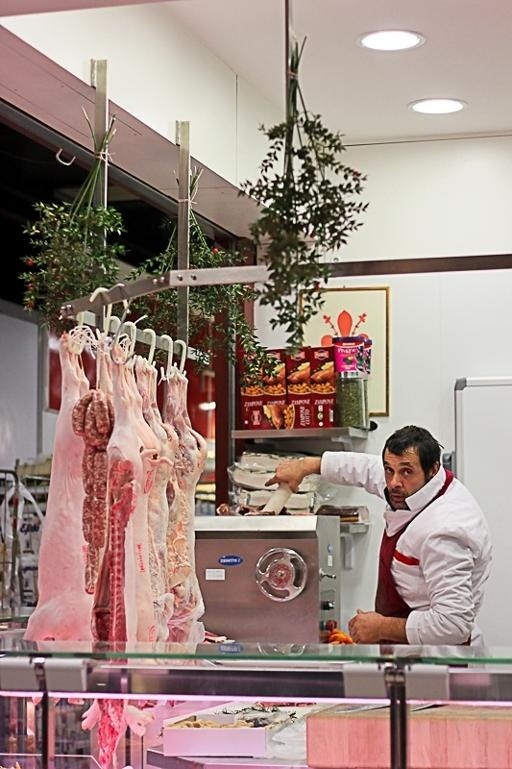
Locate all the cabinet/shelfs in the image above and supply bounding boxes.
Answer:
[229,425,370,534]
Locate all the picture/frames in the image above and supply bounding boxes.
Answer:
[298,284,391,416]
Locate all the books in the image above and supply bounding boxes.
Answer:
[316,504,369,523]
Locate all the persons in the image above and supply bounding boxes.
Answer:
[264,425,492,669]
